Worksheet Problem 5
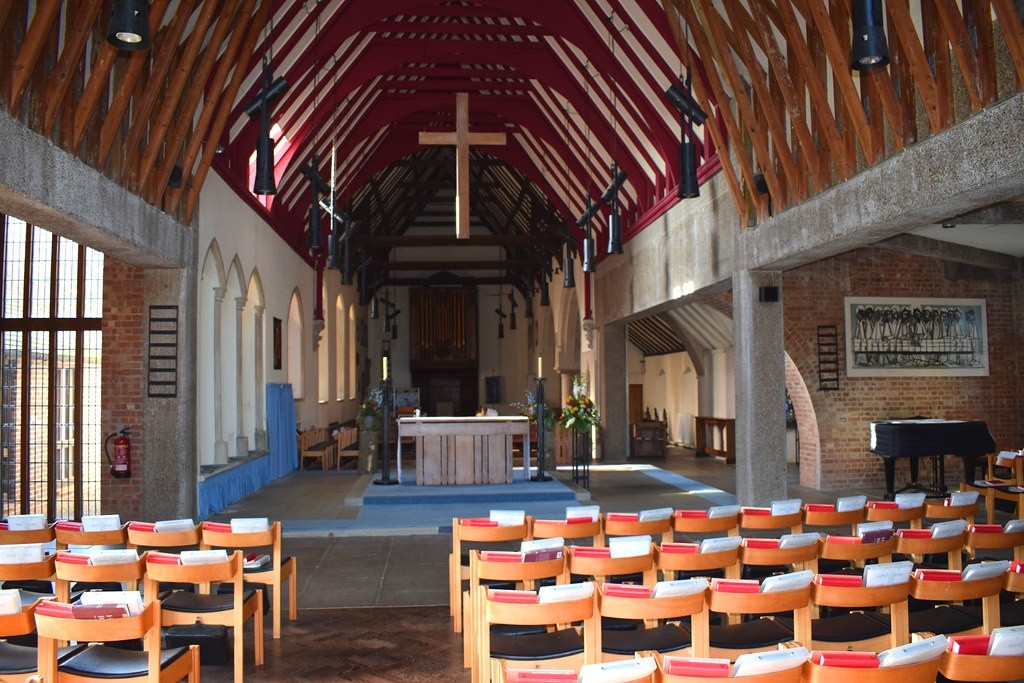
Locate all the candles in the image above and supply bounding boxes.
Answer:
[383,357,387,380]
[538,357,542,378]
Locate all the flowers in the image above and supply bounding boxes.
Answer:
[356,399,383,431]
[559,374,602,436]
[510,390,555,430]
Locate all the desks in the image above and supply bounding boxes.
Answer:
[696,416,735,465]
[396,416,531,484]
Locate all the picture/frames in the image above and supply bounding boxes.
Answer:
[844,297,989,377]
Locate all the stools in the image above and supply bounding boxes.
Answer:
[976,457,1010,480]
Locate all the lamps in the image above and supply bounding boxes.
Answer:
[496,0,709,338]
[850,0,891,71]
[107,0,148,50]
[253,0,398,339]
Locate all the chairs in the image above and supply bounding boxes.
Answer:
[448,452,1024,683]
[0,519,298,683]
[299,427,358,471]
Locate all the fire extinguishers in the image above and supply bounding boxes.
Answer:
[104,426,134,478]
